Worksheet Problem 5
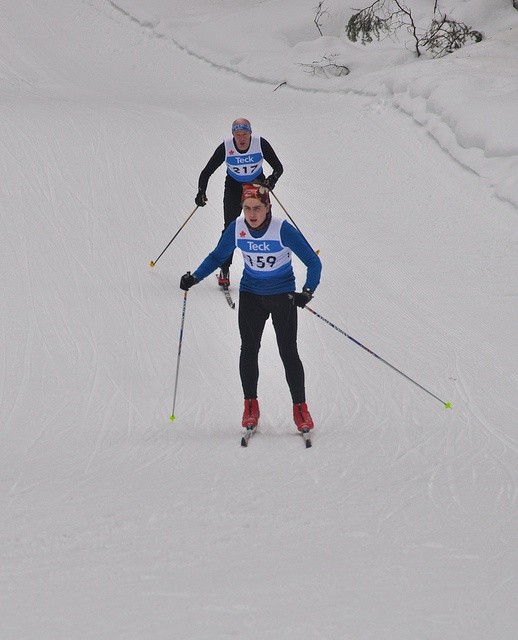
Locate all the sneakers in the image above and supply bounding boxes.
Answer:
[293,402,314,432]
[242,399,260,427]
[218,271,230,286]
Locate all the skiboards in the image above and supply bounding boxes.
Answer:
[241,427,311,448]
[215,273,235,308]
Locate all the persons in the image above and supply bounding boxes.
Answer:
[178,183,323,437]
[194,117,284,287]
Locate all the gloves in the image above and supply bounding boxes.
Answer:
[263,175,277,191]
[194,191,208,207]
[291,288,312,309]
[179,274,195,292]
